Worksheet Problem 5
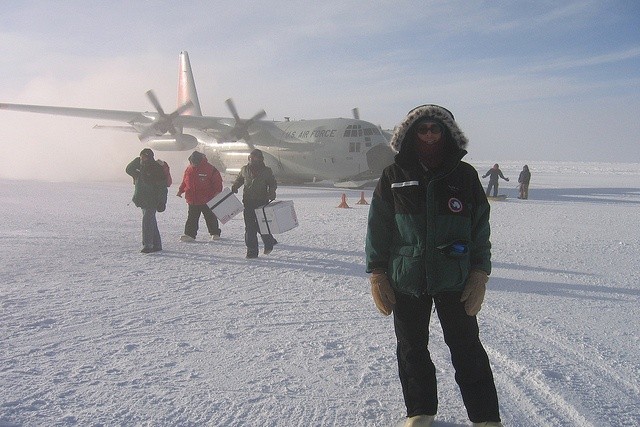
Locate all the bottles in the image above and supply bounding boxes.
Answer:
[450,242,466,254]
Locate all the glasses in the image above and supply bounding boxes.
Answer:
[417,124,442,135]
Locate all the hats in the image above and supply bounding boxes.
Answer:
[140,148,154,159]
[250,149,263,160]
[189,151,201,163]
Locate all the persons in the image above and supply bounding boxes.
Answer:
[482,163,510,197]
[517,164,531,199]
[176,151,222,242]
[365,104,503,427]
[126,148,168,253]
[232,149,277,259]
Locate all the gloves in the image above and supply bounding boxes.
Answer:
[269,192,276,199]
[369,272,396,316]
[504,178,509,181]
[482,174,486,178]
[157,204,167,212]
[461,267,488,317]
[176,191,183,198]
[232,185,238,193]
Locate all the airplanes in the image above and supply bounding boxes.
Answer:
[0,48,398,188]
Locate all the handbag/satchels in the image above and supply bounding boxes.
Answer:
[156,161,171,187]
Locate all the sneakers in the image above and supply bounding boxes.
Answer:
[212,234,219,240]
[263,239,278,254]
[141,248,163,253]
[180,234,194,243]
[471,421,505,427]
[246,247,258,258]
[402,415,436,427]
